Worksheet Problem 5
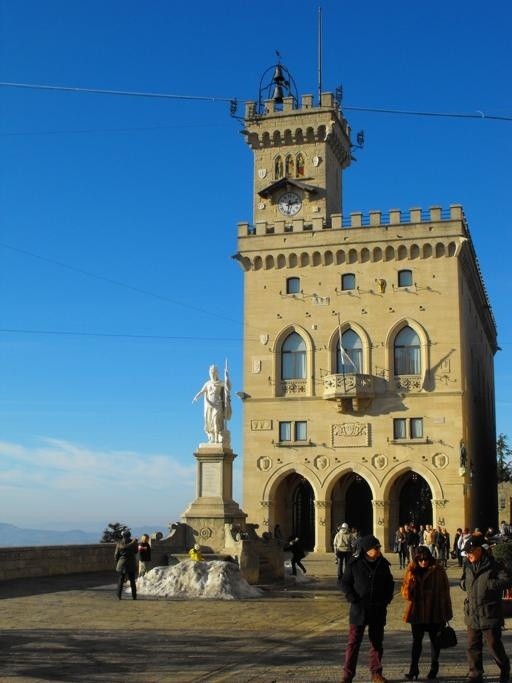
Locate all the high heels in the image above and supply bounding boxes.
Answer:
[404,666,419,680]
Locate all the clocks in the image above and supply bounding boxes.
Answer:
[277,191,302,216]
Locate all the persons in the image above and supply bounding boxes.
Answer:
[284,535,307,574]
[326,516,512,590]
[457,535,512,682]
[339,533,397,682]
[400,545,453,682]
[186,543,206,561]
[113,529,139,600]
[136,532,153,576]
[190,361,233,444]
[273,522,285,548]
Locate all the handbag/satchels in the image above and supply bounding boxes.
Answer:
[435,619,457,648]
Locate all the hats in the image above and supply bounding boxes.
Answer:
[194,544,199,550]
[458,537,481,552]
[341,522,348,527]
[360,535,378,552]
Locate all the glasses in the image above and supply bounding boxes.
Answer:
[417,557,428,560]
[465,548,476,553]
[366,545,380,550]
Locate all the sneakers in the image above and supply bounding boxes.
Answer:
[371,674,387,683]
[499,667,510,682]
